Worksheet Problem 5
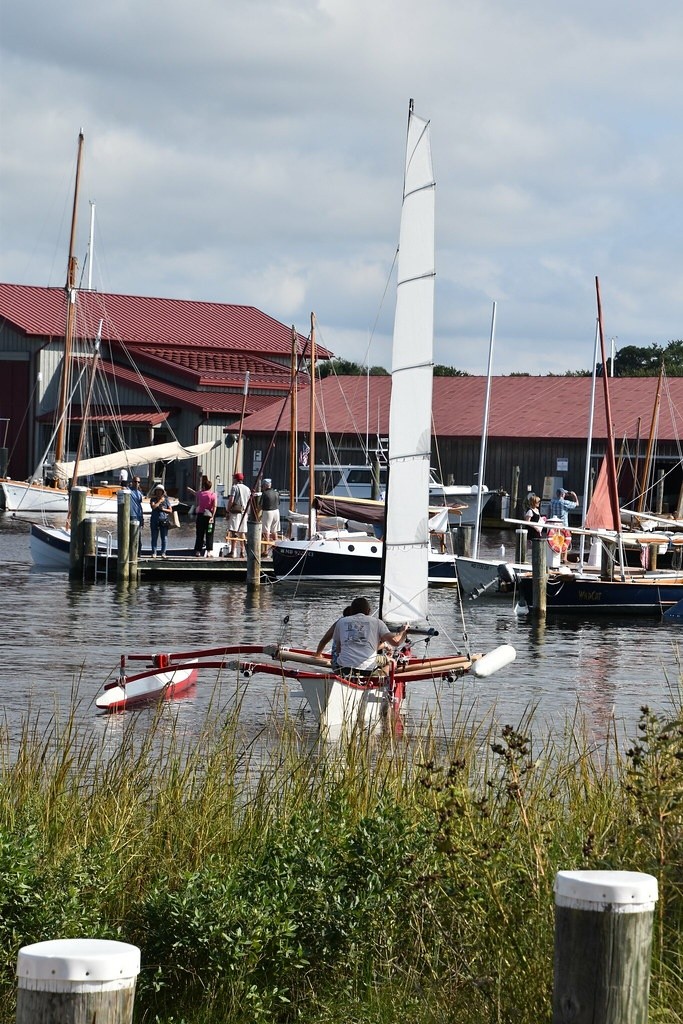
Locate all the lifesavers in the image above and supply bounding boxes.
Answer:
[545,527,573,554]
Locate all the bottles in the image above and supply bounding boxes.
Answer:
[208,524,212,533]
[219,545,229,557]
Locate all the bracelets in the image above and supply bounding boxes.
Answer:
[226,511,229,513]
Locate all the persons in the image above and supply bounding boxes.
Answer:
[150,485,172,558]
[194,480,217,558]
[224,473,251,558]
[257,481,280,557]
[525,496,542,542]
[127,477,144,557]
[119,467,128,490]
[315,598,410,686]
[548,488,579,564]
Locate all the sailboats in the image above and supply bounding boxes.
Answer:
[2,96,682,622]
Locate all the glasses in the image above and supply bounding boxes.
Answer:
[260,485,264,487]
[134,480,141,484]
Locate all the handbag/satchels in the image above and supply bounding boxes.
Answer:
[230,503,243,514]
[166,510,181,529]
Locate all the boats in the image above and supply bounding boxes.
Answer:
[291,640,420,745]
[94,657,203,712]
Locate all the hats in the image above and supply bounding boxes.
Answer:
[151,484,165,494]
[232,473,244,481]
[262,478,272,485]
[556,488,568,494]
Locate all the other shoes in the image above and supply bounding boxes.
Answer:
[161,554,166,558]
[152,554,157,558]
[196,552,201,557]
[223,552,234,558]
[206,554,213,558]
[239,551,246,558]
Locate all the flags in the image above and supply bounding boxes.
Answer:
[299,442,310,466]
